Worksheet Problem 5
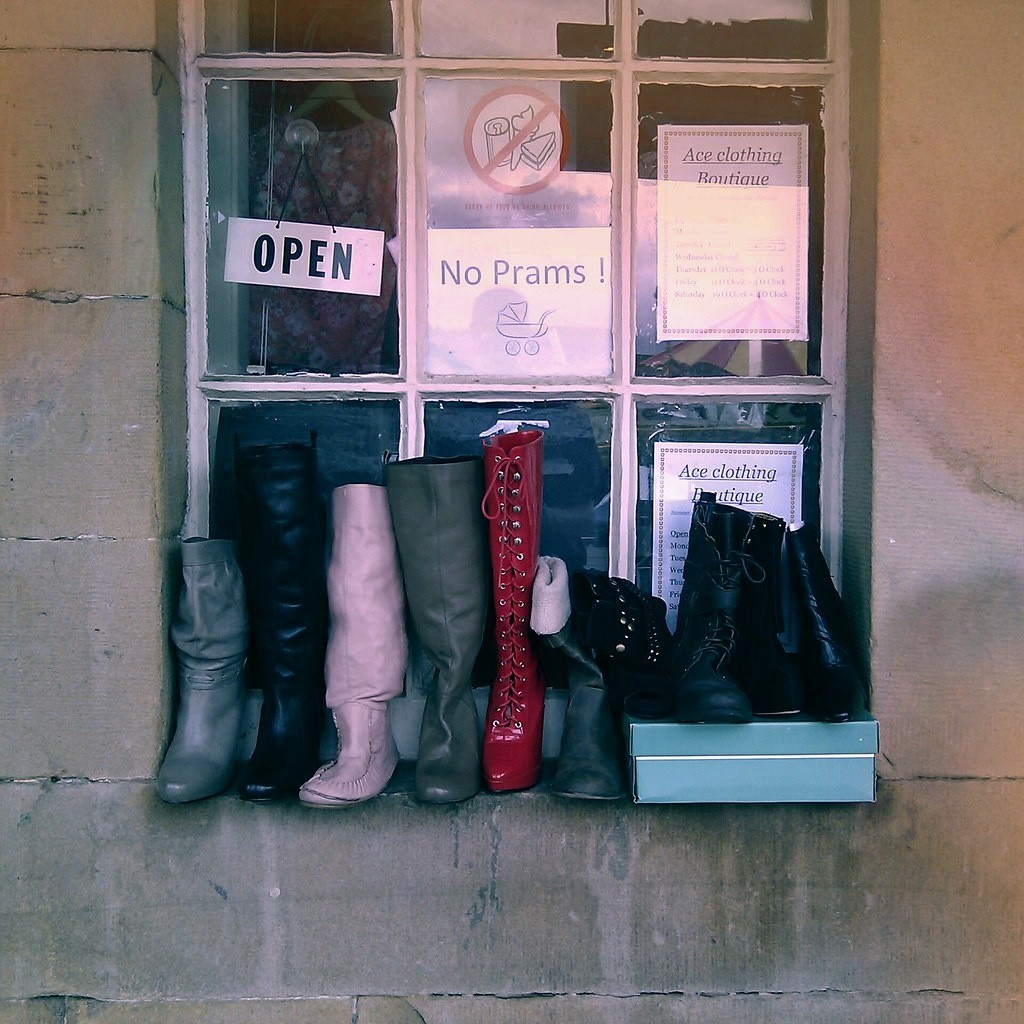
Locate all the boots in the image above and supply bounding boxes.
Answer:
[155,427,860,808]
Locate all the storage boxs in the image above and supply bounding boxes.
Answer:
[622,707,880,808]
